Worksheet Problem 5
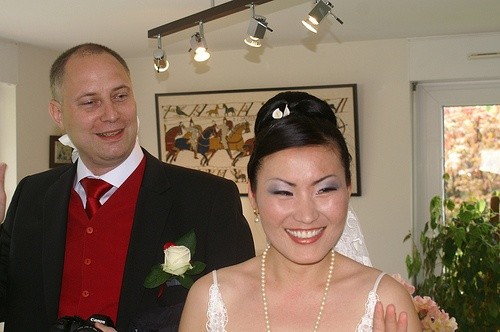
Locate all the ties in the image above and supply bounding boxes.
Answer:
[80,177,113,220]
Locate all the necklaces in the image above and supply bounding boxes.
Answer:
[261,244,335,332]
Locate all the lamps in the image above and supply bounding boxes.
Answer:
[188,20,210,62]
[302,0,344,34]
[244,2,273,48]
[152,34,169,73]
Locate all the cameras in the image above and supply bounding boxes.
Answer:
[49,314,114,332]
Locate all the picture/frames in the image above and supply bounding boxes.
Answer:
[155,84,362,196]
[49,136,74,168]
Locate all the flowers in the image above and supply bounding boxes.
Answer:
[393,274,458,332]
[144,232,207,290]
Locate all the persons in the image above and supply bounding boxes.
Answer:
[0,42,256,332]
[177,91,422,332]
